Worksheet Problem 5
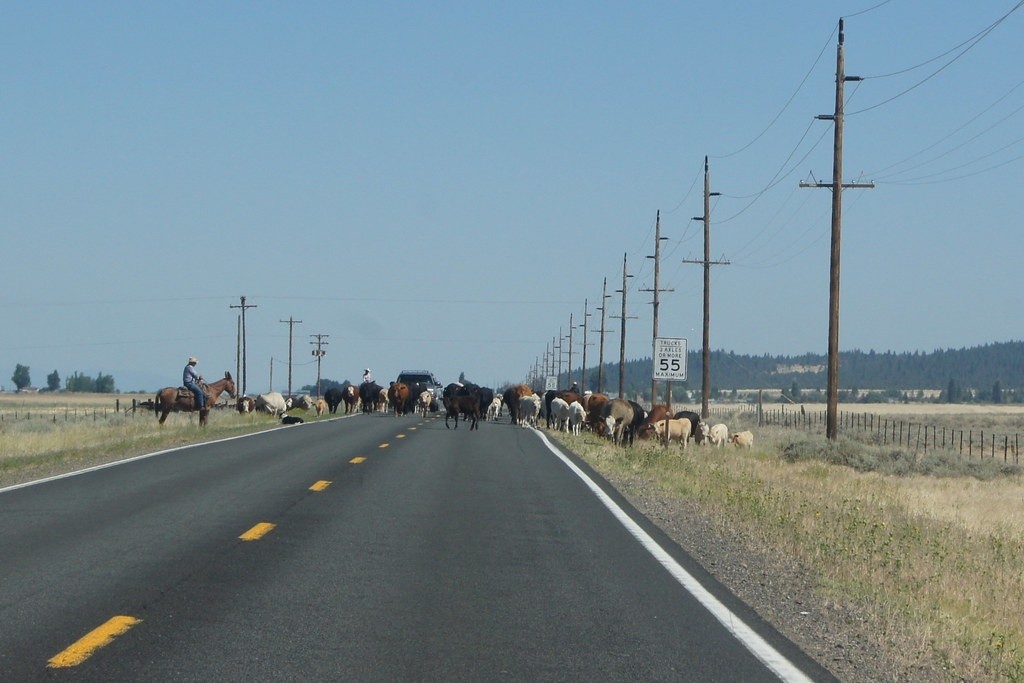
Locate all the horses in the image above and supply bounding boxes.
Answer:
[154,370,239,429]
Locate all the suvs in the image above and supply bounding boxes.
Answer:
[394,369,444,412]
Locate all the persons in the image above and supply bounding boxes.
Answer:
[183,357,207,410]
[362,367,369,383]
[570,382,580,393]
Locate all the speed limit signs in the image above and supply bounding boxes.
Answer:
[652,338,687,382]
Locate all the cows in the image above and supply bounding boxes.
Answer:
[236,380,755,451]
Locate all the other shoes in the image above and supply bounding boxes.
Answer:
[200,407,205,411]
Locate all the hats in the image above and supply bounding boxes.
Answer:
[189,357,197,363]
[573,382,577,384]
[365,368,370,372]
